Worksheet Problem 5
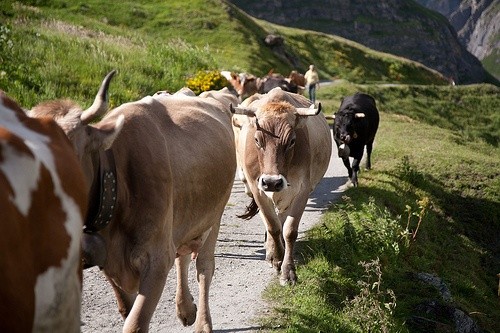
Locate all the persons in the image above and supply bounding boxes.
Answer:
[305,64,320,104]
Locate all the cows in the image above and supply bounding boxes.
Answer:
[23,70,239,333]
[1,88,86,333]
[230,86,333,288]
[151,69,307,199]
[325,93,380,188]
